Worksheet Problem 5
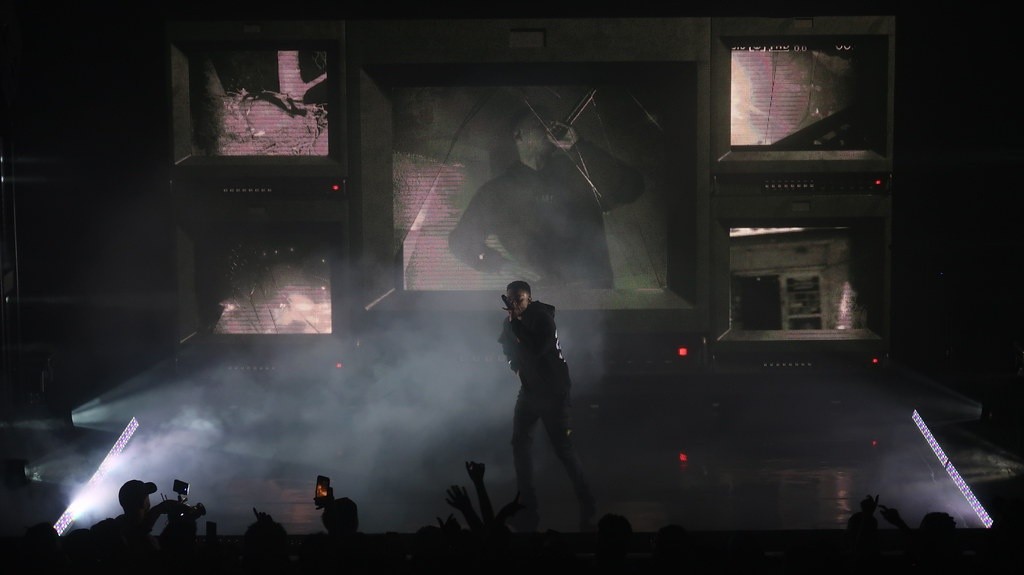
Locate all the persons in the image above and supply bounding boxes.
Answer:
[448,108,647,290]
[110,477,159,521]
[1,456,1024,574]
[498,280,595,520]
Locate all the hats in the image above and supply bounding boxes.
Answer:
[119,480,158,506]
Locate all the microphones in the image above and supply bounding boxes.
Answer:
[501,295,514,310]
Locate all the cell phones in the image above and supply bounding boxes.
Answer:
[315,475,330,498]
[206,521,216,541]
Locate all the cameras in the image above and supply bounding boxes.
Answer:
[173,479,189,495]
